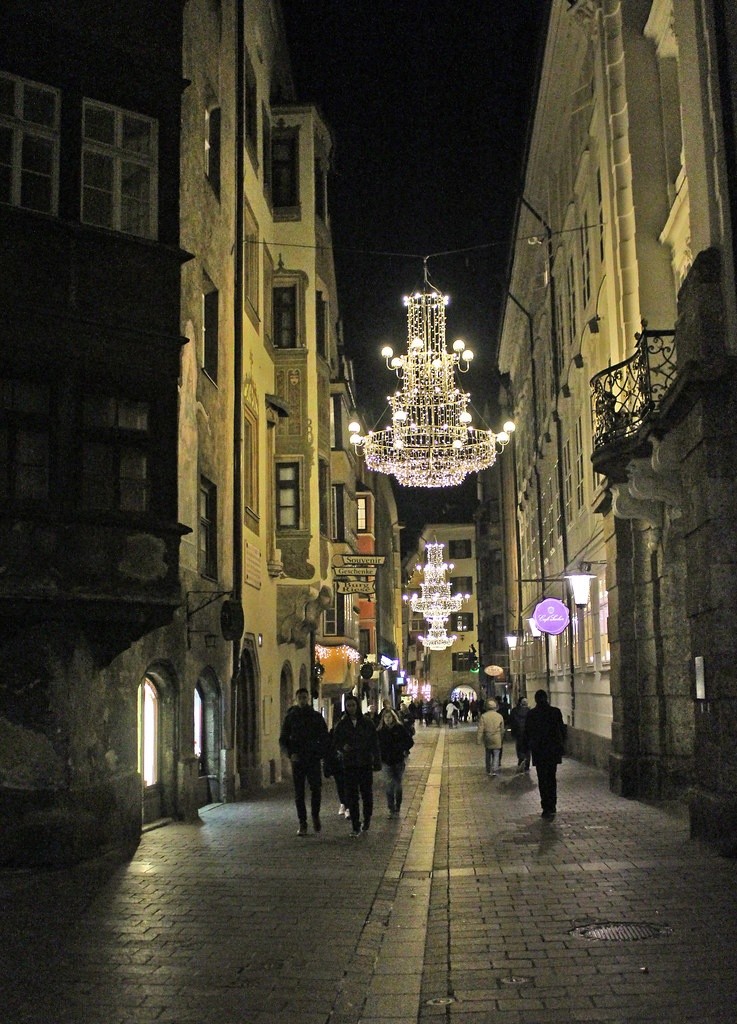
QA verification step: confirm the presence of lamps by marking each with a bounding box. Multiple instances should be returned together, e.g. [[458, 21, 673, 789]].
[[561, 358, 574, 398], [587, 274, 607, 334], [573, 319, 590, 369], [526, 617, 542, 639], [551, 391, 561, 424], [517, 491, 530, 512], [402, 536, 470, 651], [563, 559, 606, 610], [504, 630, 520, 651], [529, 436, 545, 488], [346, 257, 517, 489], [543, 424, 552, 443]]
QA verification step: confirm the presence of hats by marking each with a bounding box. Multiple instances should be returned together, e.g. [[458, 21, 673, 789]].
[[521, 698, 529, 706], [534, 689, 548, 703]]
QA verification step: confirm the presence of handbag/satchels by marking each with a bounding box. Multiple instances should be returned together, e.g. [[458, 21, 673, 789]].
[[321, 729, 334, 778], [352, 742, 374, 777], [410, 727, 416, 735]]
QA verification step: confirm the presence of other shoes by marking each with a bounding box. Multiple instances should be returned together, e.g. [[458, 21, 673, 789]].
[[311, 811, 321, 832], [296, 821, 308, 836], [543, 809, 557, 822], [349, 829, 361, 837], [393, 810, 399, 818], [386, 810, 394, 819], [362, 820, 370, 830]]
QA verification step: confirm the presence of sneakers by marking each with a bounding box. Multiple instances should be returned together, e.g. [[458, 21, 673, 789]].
[[344, 808, 351, 819], [338, 804, 345, 814]]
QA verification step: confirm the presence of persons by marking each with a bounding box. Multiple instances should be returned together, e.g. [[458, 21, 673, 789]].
[[279, 688, 329, 836], [377, 698, 415, 819], [470, 696, 511, 722], [365, 704, 378, 721], [510, 696, 531, 772], [527, 690, 565, 817], [477, 696, 508, 776], [323, 696, 382, 837], [408, 691, 469, 728]]
